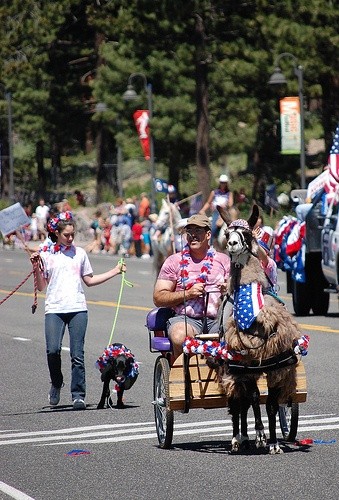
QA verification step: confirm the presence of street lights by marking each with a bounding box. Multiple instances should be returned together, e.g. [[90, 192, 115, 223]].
[[269, 52, 306, 191], [121, 72, 157, 215]]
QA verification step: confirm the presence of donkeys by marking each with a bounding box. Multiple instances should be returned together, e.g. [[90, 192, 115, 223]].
[[214, 204, 300, 456], [96, 342, 140, 409]]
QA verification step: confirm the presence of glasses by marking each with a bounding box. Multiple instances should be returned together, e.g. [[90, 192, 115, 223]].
[[185, 229, 204, 234]]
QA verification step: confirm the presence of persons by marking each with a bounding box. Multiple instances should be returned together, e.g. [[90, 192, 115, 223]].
[[89, 188, 189, 258], [198, 175, 233, 243], [153, 214, 231, 358], [30, 211, 127, 409], [35, 196, 50, 238]]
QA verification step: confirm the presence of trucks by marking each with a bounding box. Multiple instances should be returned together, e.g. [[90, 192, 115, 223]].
[[291, 168, 339, 315]]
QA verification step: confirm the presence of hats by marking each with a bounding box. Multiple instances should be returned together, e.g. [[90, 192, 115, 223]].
[[219, 175, 228, 182], [184, 215, 212, 231]]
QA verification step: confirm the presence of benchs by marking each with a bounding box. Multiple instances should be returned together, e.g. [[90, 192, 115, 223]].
[[146, 306, 172, 352]]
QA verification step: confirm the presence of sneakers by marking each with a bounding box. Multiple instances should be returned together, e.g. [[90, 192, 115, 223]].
[[48, 382, 65, 405], [73, 399, 86, 409]]
